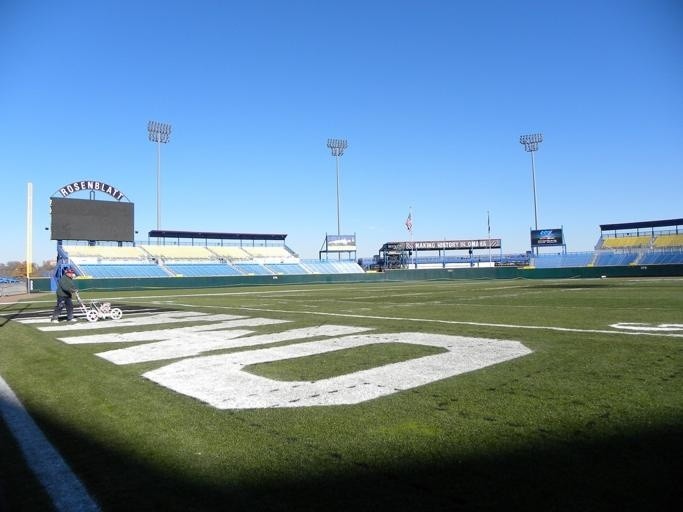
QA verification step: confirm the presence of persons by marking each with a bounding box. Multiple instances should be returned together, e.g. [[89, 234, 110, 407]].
[[51, 269, 77, 323]]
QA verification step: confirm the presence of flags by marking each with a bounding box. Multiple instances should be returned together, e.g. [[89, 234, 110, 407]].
[[405, 212, 413, 230]]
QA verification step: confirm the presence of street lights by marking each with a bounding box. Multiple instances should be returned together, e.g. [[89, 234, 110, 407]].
[[518, 133, 545, 255], [325, 138, 350, 236], [146, 121, 172, 244]]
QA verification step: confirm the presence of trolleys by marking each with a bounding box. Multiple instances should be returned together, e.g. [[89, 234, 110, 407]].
[[72, 289, 122, 321]]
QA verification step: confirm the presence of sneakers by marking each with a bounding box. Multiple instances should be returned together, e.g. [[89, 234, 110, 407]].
[[51, 320, 60, 323], [71, 319, 78, 322]]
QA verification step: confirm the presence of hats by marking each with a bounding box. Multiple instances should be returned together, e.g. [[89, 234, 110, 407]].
[[65, 268, 75, 273]]
